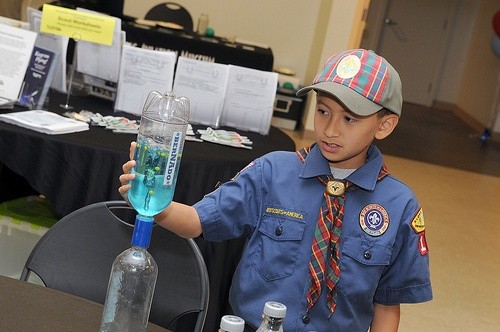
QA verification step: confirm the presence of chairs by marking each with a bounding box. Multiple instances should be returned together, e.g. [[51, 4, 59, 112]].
[[17, 199, 211, 329], [141, 2, 195, 32]]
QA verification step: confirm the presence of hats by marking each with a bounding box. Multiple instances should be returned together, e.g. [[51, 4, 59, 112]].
[[295, 47, 404, 117]]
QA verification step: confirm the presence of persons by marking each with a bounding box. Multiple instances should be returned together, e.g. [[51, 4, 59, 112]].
[[117, 47, 434, 332]]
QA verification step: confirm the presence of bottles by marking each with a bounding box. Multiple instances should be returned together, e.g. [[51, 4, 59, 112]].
[[128, 89, 190, 235], [99, 225, 159, 332], [256, 300, 286, 332], [218, 315, 245, 332]]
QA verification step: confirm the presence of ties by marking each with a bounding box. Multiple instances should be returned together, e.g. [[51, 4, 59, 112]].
[[295, 146, 392, 321]]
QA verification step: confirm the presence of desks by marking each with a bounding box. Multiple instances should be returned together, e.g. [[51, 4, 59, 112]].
[[105, 10, 275, 73], [1, 84, 299, 234], [0, 274, 179, 332]]
